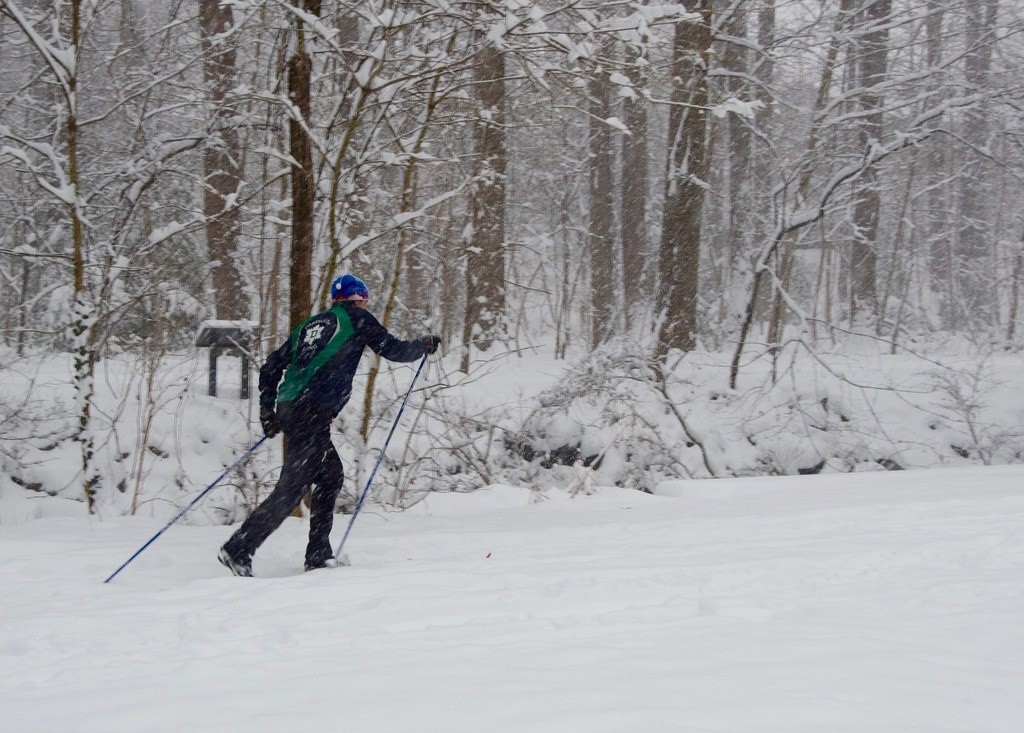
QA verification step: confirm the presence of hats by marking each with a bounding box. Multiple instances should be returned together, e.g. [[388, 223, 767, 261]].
[[330, 275, 368, 303]]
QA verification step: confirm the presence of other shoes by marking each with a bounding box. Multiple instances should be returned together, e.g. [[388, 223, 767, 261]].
[[218, 547, 254, 578], [304, 557, 351, 572]]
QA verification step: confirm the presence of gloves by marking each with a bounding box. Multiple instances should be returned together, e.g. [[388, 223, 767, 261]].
[[422, 334, 442, 356], [263, 418, 280, 439]]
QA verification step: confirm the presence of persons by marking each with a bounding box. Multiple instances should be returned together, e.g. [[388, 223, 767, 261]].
[[217, 274, 442, 577]]
